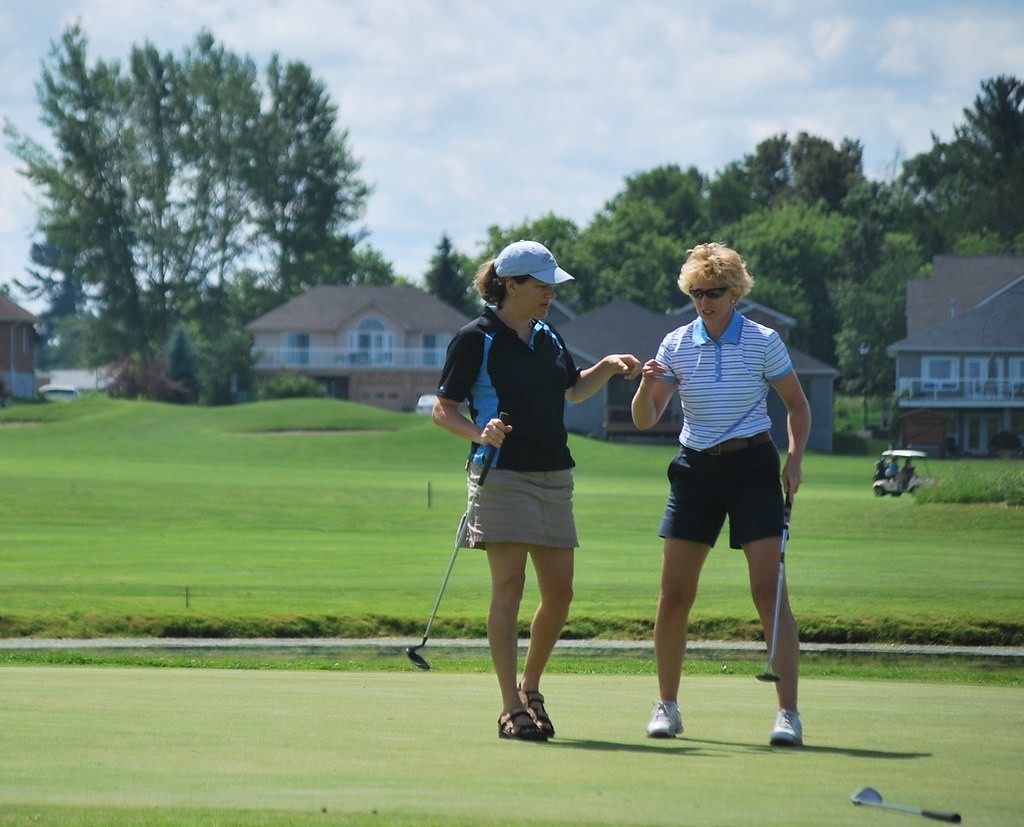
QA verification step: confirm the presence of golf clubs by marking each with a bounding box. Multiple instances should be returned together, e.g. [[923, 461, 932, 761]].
[[852, 787, 962, 824], [754, 491, 791, 683], [405, 412, 510, 670]]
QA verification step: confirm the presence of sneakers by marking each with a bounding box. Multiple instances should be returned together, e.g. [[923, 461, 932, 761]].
[[646, 699, 684, 739], [770, 710, 801, 746]]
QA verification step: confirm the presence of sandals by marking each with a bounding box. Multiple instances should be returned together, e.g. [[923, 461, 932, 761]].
[[517, 684, 555, 737], [497, 711, 545, 740]]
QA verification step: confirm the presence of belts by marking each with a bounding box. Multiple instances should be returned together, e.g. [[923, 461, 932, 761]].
[[702, 431, 772, 456]]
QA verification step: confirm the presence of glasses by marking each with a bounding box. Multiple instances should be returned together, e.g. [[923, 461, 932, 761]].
[[689, 287, 728, 300]]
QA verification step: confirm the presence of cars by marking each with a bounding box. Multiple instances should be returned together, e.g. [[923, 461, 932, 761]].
[[37, 383, 81, 402], [415, 393, 435, 414]]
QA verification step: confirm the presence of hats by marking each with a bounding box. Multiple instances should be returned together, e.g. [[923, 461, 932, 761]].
[[493, 240, 575, 284]]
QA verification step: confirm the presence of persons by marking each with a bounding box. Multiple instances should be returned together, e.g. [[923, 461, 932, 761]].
[[902, 458, 912, 473], [875, 457, 899, 481], [631, 242, 811, 747], [432, 240, 642, 742]]
